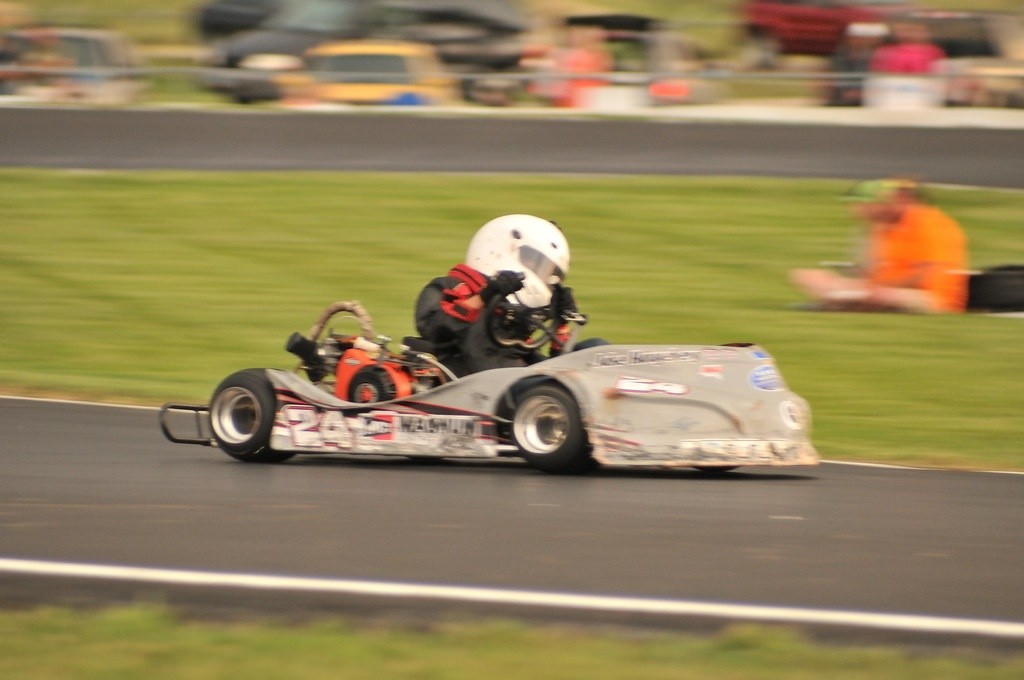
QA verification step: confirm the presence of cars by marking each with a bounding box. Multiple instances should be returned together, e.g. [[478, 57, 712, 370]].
[[1, 2, 1023, 114]]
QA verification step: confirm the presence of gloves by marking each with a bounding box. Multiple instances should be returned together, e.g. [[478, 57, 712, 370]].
[[550, 285, 577, 325], [479, 270, 523, 306]]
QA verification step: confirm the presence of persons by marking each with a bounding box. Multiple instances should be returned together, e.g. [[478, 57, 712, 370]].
[[826, 19, 947, 108], [789, 178, 1024, 314], [519, 25, 615, 107], [414, 213, 611, 387]]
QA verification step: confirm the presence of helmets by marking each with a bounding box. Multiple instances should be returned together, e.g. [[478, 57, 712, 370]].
[[465, 214, 569, 308]]
